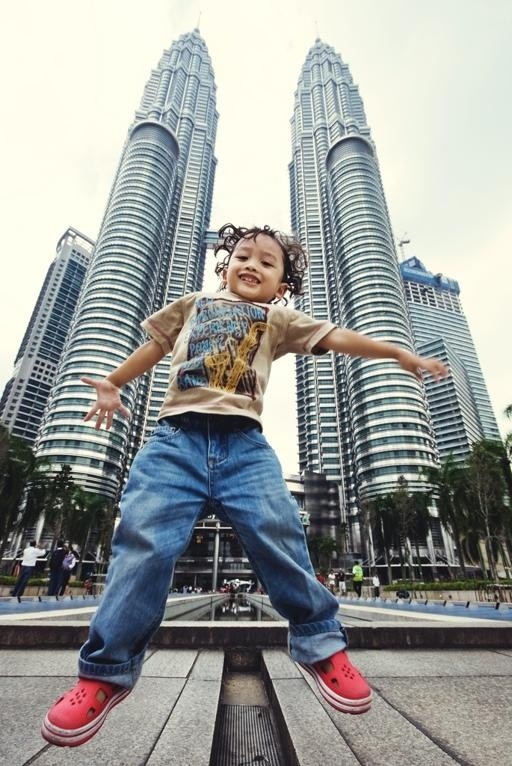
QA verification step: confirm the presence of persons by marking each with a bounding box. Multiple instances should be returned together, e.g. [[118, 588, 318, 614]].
[[317, 560, 346, 595], [10, 539, 93, 597], [352, 561, 364, 597], [172, 580, 241, 595], [43, 221, 446, 748], [372, 573, 380, 598]]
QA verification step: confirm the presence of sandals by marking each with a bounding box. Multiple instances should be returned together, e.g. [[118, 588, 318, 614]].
[[41, 679, 130, 747], [296, 654, 373, 714]]
